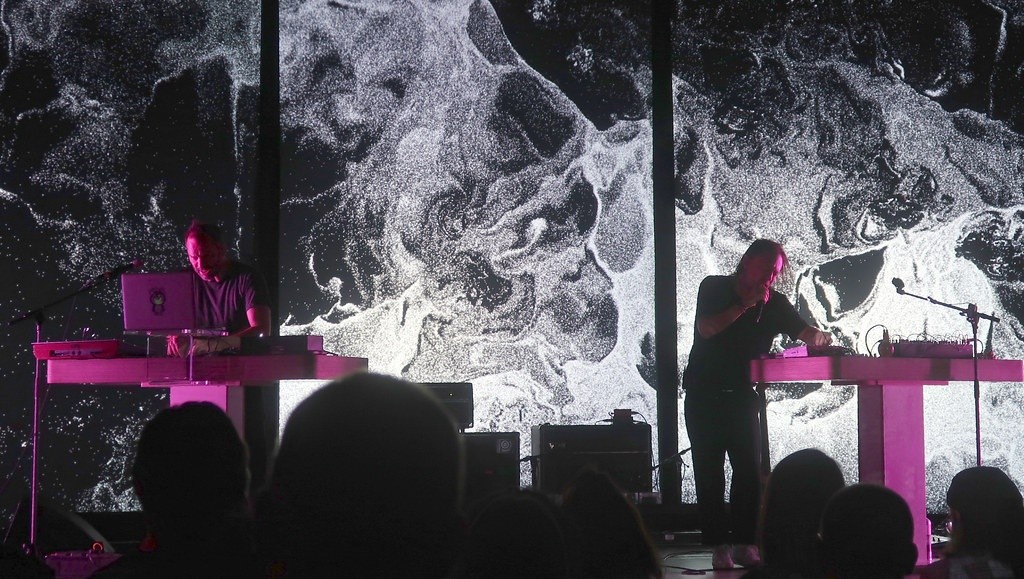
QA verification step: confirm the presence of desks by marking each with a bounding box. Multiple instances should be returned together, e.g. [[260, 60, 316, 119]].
[[49, 353, 369, 432], [751, 356, 1024, 567]]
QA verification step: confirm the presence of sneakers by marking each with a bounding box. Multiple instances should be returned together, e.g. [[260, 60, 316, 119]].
[[712, 544, 760, 569]]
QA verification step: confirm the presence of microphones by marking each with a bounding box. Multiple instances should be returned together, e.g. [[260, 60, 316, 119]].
[[753, 301, 764, 322], [97, 259, 142, 280]]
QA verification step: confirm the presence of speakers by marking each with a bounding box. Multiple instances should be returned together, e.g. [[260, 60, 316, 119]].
[[463, 431, 520, 502], [532, 425, 653, 497]]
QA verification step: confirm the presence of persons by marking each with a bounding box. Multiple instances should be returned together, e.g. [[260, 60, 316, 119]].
[[0, 372, 1024, 579], [166, 221, 272, 494], [682, 240, 833, 570]]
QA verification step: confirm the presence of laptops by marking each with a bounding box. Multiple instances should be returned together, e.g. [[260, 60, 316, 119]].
[[122, 272, 229, 338]]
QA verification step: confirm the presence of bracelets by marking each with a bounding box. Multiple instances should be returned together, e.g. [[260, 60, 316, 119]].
[[734, 299, 749, 312]]
